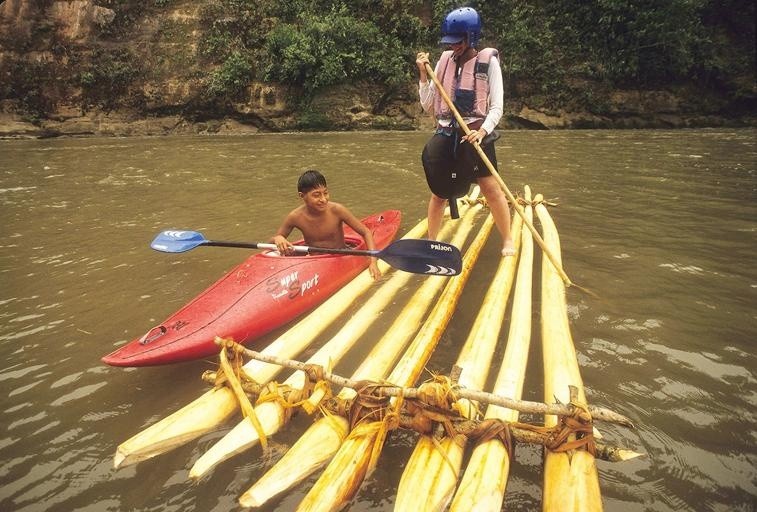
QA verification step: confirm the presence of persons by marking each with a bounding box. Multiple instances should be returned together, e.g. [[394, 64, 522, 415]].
[[415, 5, 517, 257], [266, 169, 383, 282]]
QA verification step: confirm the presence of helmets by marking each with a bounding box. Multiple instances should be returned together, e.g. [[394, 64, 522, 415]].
[[439, 7, 481, 48]]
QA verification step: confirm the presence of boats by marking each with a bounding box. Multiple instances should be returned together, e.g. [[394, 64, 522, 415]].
[[114, 180, 643, 511], [101, 211, 402, 366]]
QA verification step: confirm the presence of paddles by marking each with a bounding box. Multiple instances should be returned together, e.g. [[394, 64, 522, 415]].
[[151, 229, 462, 275]]
